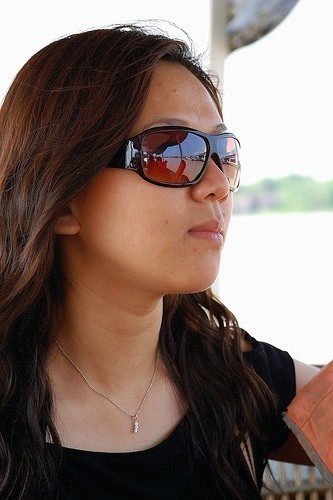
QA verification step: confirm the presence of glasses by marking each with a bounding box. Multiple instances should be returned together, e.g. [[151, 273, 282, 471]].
[[104, 125, 242, 192]]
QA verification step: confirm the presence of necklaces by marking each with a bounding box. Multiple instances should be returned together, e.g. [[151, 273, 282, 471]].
[[52, 334, 160, 434]]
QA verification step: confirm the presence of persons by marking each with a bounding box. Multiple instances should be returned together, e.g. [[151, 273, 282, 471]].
[[1, 19, 333, 500]]
